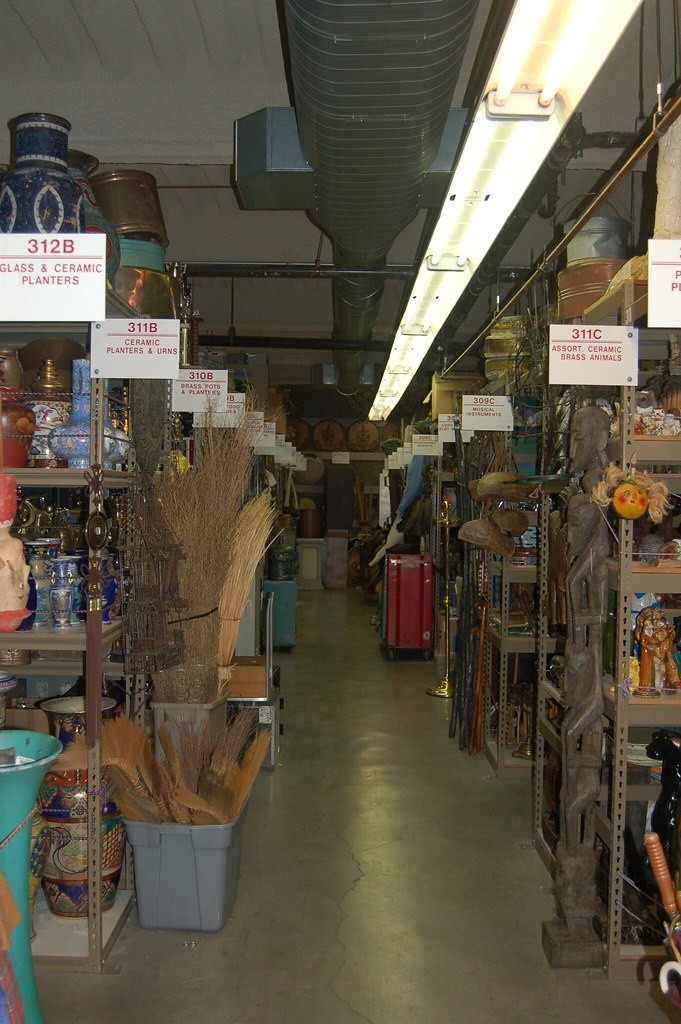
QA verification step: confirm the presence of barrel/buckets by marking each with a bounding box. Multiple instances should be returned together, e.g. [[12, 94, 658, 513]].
[[563, 192, 633, 267], [89, 169, 169, 249]]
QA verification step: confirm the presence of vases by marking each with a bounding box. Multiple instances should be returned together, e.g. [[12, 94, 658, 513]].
[[0, 113, 84, 235], [0, 347, 128, 1024], [65, 150, 119, 283]]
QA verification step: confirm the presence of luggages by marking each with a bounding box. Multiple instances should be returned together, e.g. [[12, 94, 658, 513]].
[[380, 546, 433, 661]]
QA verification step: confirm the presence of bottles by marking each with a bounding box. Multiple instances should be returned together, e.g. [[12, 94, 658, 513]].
[[25, 538, 117, 629], [0, 112, 120, 280]]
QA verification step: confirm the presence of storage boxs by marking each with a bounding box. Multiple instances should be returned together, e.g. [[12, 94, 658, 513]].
[[225, 656, 266, 698], [122, 789, 247, 932], [263, 581, 295, 647], [430, 369, 481, 422], [150, 691, 227, 763], [4, 696, 53, 735]]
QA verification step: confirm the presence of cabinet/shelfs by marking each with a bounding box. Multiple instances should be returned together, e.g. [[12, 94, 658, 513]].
[[0, 324, 172, 972], [427, 277, 681, 982]]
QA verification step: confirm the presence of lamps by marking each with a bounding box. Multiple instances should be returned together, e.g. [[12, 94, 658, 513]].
[[368, 0, 646, 421]]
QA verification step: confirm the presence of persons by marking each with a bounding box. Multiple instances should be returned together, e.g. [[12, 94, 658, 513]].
[[543, 406, 610, 968], [634, 406, 681, 435], [638, 616, 681, 694]]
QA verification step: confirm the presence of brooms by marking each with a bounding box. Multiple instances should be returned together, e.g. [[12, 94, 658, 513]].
[[46, 705, 275, 827], [136, 385, 285, 702]]
